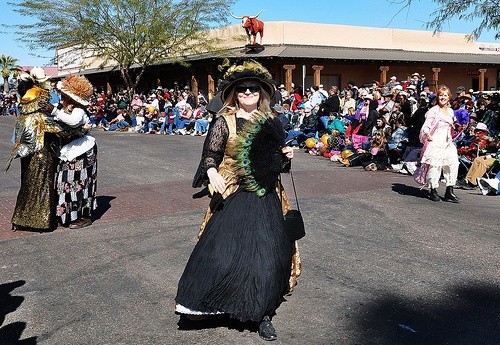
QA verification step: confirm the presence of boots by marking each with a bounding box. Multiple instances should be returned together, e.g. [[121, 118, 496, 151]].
[[430, 188, 441, 201], [444, 186, 459, 202]]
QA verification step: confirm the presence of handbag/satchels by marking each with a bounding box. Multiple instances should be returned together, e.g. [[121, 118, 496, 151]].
[[285, 209, 306, 241]]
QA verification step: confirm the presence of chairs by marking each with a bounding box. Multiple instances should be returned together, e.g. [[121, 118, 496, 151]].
[[459, 144, 497, 179]]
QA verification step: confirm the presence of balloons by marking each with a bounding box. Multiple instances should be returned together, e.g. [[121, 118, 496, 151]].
[[342, 149, 351, 158], [321, 133, 330, 146], [306, 138, 316, 149], [148, 106, 155, 113]]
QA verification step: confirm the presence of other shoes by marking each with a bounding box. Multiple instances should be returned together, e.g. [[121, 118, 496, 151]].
[[476, 177, 499, 196], [258, 315, 277, 341], [453, 178, 476, 190], [391, 164, 409, 175], [69, 217, 93, 229], [134, 128, 208, 137], [337, 157, 350, 167]]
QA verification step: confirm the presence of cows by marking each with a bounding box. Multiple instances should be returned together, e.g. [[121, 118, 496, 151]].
[[229, 10, 264, 47]]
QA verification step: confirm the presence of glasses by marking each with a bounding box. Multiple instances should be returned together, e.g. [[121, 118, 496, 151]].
[[421, 76, 425, 78], [236, 85, 260, 93]]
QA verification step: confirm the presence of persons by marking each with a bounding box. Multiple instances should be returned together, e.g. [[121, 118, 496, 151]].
[[174, 60, 294, 341], [0, 74, 500, 229]]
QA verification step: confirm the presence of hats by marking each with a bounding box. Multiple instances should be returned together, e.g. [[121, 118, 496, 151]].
[[456, 85, 471, 99], [474, 122, 491, 135], [216, 57, 275, 106], [470, 112, 477, 119], [277, 72, 427, 129], [56, 74, 93, 106], [19, 86, 50, 114], [150, 86, 191, 100]]
